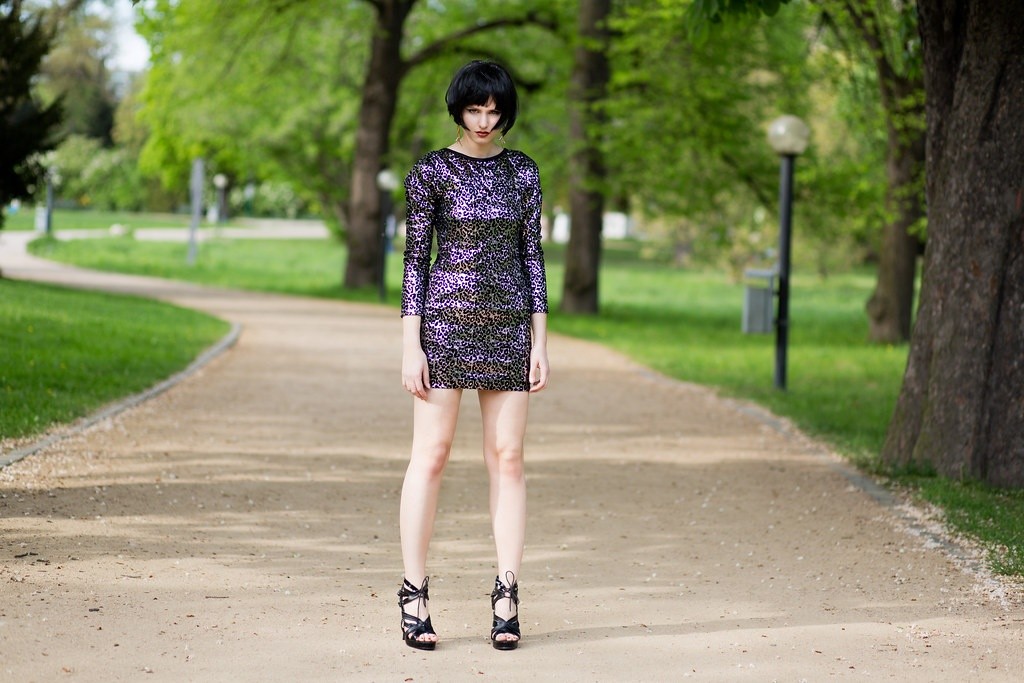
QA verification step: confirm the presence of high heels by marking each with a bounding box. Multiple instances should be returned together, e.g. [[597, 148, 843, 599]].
[[490, 570, 521, 649], [397, 576, 438, 650]]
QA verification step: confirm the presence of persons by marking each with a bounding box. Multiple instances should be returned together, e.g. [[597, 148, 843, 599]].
[[396, 61, 550, 651]]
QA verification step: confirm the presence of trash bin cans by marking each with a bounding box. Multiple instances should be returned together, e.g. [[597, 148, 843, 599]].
[[743, 270, 777, 335]]
